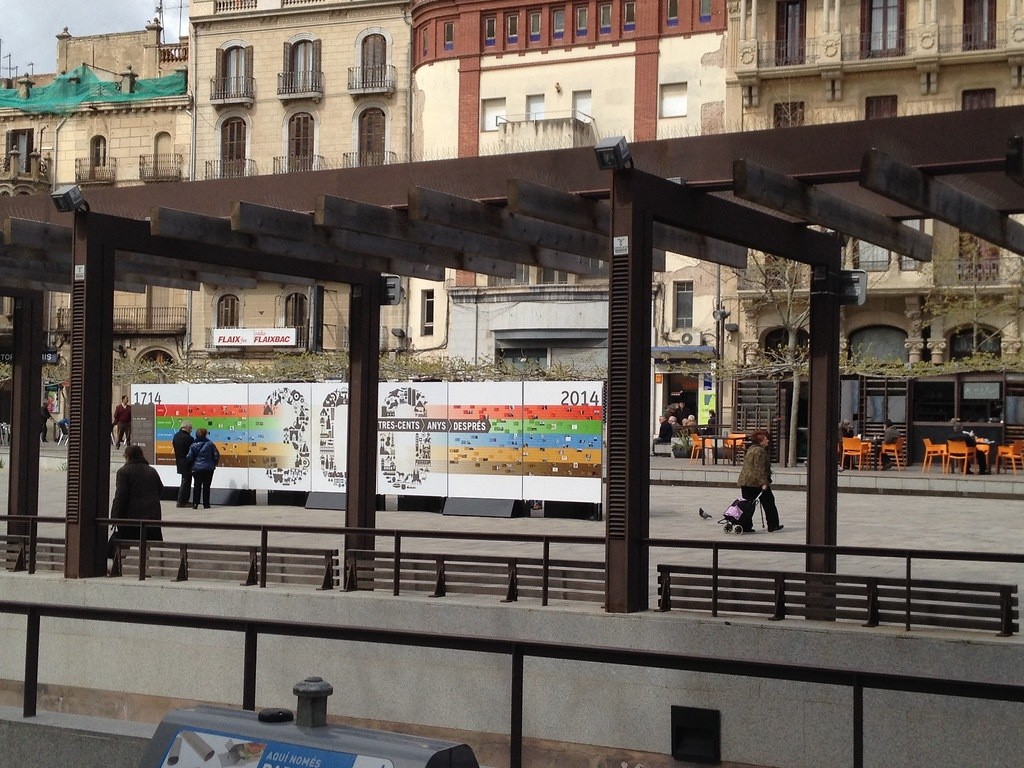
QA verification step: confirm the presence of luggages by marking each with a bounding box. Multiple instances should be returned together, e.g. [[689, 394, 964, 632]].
[[718, 489, 768, 535]]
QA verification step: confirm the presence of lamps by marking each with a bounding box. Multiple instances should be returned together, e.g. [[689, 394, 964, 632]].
[[50, 185, 90, 213], [725, 323, 739, 332], [594, 135, 634, 171], [391, 328, 405, 339]]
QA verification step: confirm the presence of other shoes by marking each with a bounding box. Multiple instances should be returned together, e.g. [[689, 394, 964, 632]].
[[178, 502, 193, 507], [966, 470, 974, 475], [844, 464, 858, 469], [193, 503, 198, 509], [116, 443, 120, 449], [881, 462, 894, 471], [203, 504, 210, 508], [978, 471, 989, 475]]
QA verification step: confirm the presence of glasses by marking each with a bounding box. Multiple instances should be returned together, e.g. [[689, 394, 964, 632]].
[[123, 454, 128, 458]]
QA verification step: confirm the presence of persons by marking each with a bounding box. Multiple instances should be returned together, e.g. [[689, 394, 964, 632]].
[[111, 445, 166, 578], [738, 430, 784, 532], [873, 420, 900, 470], [113, 395, 131, 450], [949, 421, 989, 476], [172, 422, 198, 508], [40, 402, 57, 442], [186, 428, 220, 508], [837, 419, 858, 470], [650, 402, 715, 455]]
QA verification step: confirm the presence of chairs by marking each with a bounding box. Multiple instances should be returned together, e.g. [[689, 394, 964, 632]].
[[690, 433, 713, 465], [995, 440, 1024, 476], [946, 440, 977, 475], [878, 438, 906, 471], [921, 438, 948, 474], [841, 437, 869, 471], [722, 434, 747, 465], [57, 430, 69, 446]]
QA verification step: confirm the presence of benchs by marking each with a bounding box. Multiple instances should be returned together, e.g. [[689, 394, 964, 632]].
[[340, 548, 606, 610], [107, 539, 339, 591], [653, 563, 1019, 638], [0, 535, 65, 573]]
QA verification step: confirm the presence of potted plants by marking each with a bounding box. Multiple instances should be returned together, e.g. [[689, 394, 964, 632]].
[[671, 428, 693, 459]]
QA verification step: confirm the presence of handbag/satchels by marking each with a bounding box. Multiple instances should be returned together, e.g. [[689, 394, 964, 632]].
[[182, 459, 195, 477], [106, 526, 121, 559]]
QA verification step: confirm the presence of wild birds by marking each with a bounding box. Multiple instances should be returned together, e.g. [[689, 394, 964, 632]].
[[699, 508, 712, 520]]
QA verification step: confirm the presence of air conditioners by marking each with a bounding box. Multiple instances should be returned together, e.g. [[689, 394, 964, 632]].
[[680, 330, 703, 346]]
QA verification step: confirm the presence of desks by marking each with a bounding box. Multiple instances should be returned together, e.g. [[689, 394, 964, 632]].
[[698, 436, 749, 466], [846, 439, 885, 471], [958, 444, 991, 474]]
